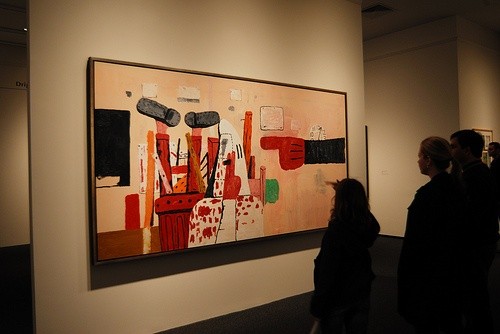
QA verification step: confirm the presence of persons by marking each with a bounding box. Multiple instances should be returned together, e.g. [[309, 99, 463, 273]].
[[309, 177, 380, 334], [447, 129, 494, 333], [395, 135, 468, 334], [487, 142, 500, 180]]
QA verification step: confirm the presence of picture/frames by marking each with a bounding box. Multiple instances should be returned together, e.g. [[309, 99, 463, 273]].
[[472, 128, 494, 170], [86, 56, 349, 267]]
